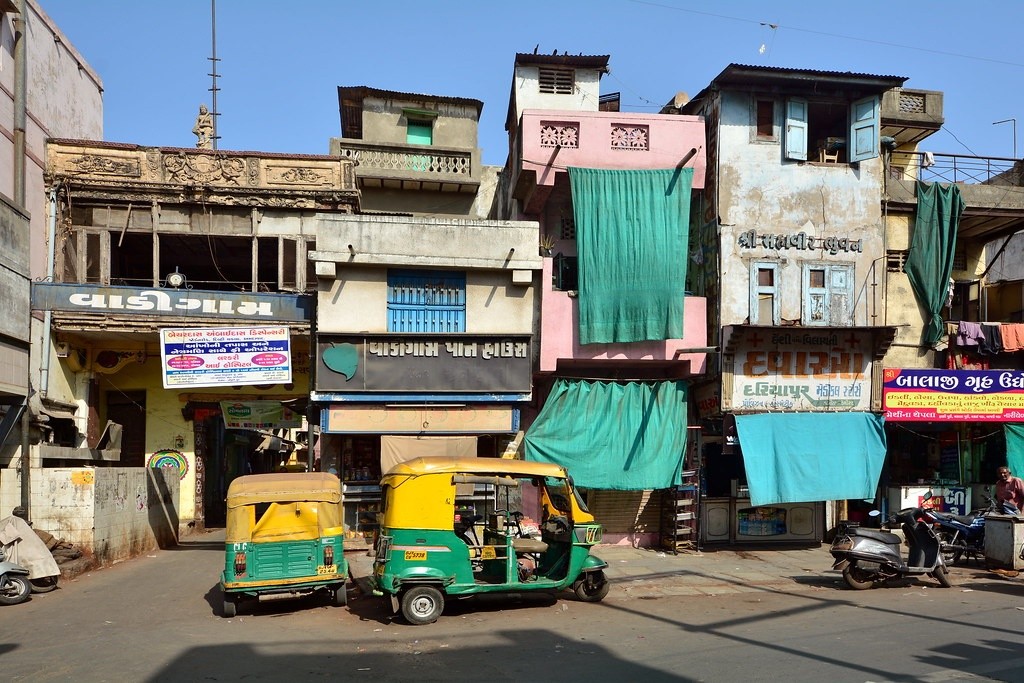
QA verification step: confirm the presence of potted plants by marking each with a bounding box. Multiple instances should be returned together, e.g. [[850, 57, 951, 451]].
[[539, 233, 558, 258]]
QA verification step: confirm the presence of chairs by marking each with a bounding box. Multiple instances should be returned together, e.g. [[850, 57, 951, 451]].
[[490, 513, 548, 580]]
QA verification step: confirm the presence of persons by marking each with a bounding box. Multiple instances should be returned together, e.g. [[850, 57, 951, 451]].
[[192, 105, 214, 145], [996, 466, 1024, 515]]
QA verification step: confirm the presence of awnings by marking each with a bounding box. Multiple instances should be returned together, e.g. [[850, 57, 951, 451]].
[[721, 324, 911, 359], [714, 63, 909, 95]]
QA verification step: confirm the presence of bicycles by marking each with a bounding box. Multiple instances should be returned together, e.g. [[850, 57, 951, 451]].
[[454, 509, 541, 570]]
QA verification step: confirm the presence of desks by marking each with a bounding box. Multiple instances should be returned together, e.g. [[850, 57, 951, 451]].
[[983, 516, 1024, 571], [888, 485, 971, 517]]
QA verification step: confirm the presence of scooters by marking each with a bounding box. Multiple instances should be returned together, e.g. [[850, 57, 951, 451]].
[[829, 491, 951, 590]]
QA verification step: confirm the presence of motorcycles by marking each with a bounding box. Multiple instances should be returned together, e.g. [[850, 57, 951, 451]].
[[924, 486, 1019, 566], [0, 516, 58, 605], [373, 457, 610, 625], [220, 472, 350, 617]]
